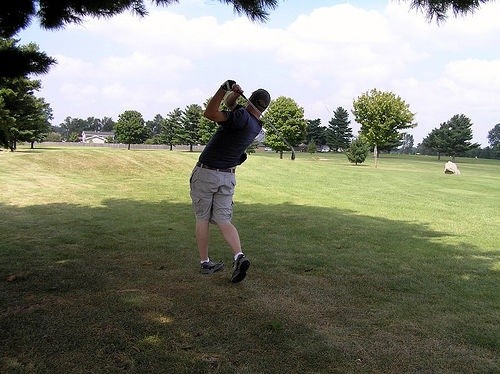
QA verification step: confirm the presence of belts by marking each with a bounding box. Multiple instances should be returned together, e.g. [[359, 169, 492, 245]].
[[196, 162, 236, 173]]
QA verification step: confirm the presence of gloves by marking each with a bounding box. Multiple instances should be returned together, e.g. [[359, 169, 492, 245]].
[[221, 79, 236, 91]]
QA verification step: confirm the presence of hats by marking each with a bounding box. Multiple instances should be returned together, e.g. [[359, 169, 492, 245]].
[[252, 89, 271, 109]]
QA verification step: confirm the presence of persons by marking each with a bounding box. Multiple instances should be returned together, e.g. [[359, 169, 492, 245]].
[[189, 80, 271, 282]]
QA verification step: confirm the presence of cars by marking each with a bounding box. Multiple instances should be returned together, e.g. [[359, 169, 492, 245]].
[[317, 146, 344, 153]]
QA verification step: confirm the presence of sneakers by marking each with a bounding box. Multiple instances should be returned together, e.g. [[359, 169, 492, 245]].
[[201, 260, 224, 274], [231, 255, 251, 283]]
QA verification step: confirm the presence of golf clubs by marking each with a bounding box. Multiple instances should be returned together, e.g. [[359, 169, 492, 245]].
[[239, 90, 295, 161]]
